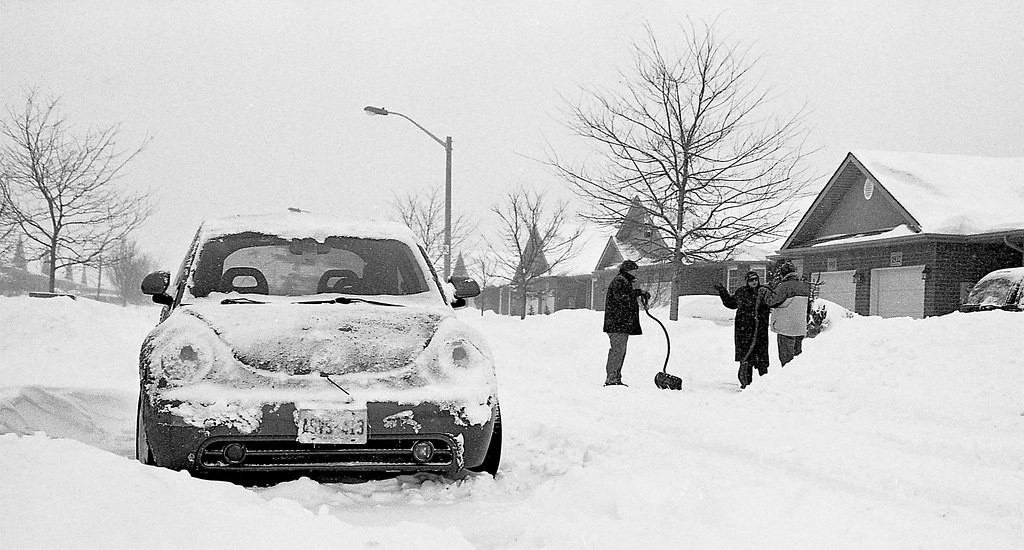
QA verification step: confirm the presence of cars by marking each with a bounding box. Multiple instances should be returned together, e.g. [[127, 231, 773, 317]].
[[135, 210, 501, 480]]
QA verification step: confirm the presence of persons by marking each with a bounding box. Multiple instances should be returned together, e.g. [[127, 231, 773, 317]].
[[603, 260, 651, 387], [757, 259, 810, 367], [712, 271, 778, 388]]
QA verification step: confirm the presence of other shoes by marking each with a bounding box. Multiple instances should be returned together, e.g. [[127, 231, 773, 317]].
[[605, 381, 628, 387]]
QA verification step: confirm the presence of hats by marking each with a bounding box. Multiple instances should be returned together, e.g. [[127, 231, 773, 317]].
[[780, 262, 796, 276], [620, 260, 638, 271], [746, 271, 758, 283]]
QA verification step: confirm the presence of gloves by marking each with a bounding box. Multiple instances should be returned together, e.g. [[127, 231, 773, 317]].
[[714, 283, 728, 294], [755, 285, 763, 291], [638, 287, 651, 299]]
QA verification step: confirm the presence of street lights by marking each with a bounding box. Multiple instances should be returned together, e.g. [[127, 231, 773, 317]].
[[363, 106, 455, 284]]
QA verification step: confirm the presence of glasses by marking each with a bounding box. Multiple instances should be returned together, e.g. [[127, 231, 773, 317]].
[[747, 276, 759, 282]]
